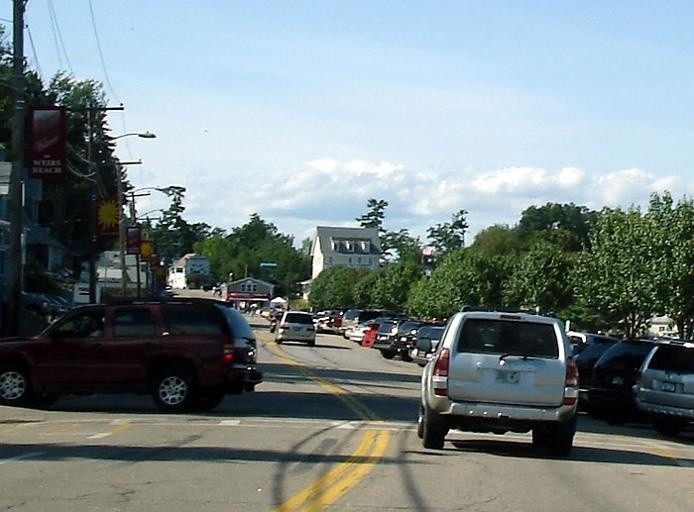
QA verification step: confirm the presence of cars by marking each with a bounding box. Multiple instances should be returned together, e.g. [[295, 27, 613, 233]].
[[1, 297, 263, 413]]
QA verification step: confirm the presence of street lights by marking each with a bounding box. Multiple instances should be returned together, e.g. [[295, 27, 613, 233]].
[[112, 132, 158, 140]]
